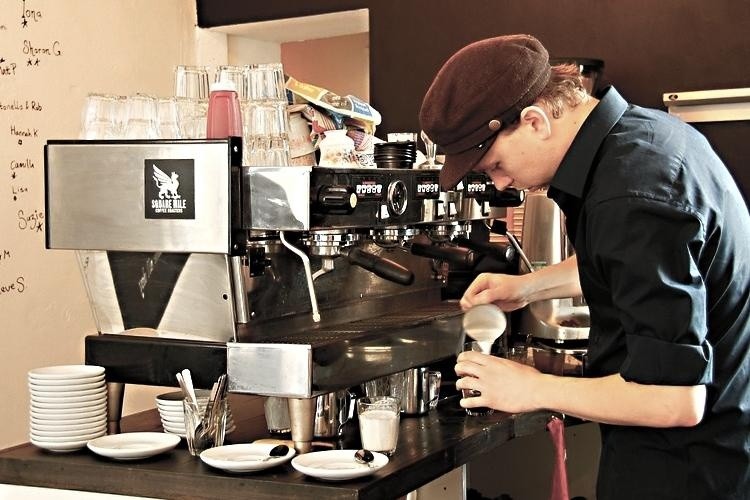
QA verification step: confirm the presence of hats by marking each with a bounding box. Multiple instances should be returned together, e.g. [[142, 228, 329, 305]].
[[418, 32, 553, 192]]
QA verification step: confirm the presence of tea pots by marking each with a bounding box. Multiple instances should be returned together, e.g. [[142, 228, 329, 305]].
[[455, 300, 511, 363]]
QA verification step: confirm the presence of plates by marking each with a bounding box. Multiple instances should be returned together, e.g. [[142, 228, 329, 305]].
[[26, 364, 109, 454], [199, 443, 296, 473], [290, 448, 388, 485], [82, 430, 181, 463], [155, 389, 237, 441]]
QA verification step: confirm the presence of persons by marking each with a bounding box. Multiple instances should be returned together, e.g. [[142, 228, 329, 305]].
[[418, 34, 749, 500]]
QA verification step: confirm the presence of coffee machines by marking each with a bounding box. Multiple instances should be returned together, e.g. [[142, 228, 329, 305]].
[[43, 139, 528, 427], [518, 190, 592, 345]]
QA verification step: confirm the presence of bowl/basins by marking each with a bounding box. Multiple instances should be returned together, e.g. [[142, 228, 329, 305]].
[[373, 141, 418, 170]]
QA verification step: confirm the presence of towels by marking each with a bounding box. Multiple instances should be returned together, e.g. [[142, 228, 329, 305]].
[[547, 417, 570, 500]]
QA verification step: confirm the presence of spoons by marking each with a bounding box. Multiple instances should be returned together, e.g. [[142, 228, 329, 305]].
[[354, 447, 378, 471], [175, 366, 229, 457], [262, 443, 288, 462]]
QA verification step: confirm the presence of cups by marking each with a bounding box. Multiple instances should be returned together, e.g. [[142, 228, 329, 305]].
[[314, 388, 356, 439], [458, 341, 508, 417], [358, 367, 443, 416], [182, 398, 227, 458], [355, 394, 401, 459], [81, 61, 363, 170], [260, 395, 292, 435]]
